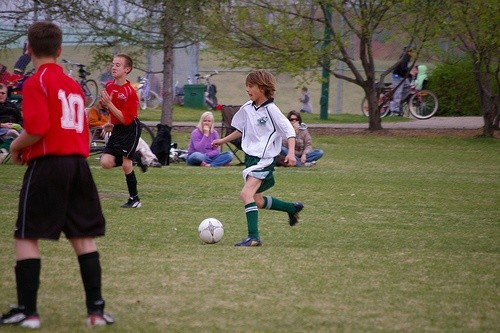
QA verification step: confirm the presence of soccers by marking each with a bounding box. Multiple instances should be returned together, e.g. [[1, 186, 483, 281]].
[[197, 217, 224, 244]]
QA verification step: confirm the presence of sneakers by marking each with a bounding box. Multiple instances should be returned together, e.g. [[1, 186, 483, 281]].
[[135, 151, 147, 171], [121, 196, 140, 209], [288, 201, 303, 225], [235, 236, 261, 247]]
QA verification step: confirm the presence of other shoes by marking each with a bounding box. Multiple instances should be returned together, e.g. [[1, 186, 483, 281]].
[[85, 313, 114, 328], [0, 308, 40, 329], [149, 160, 162, 167], [200, 161, 206, 166]]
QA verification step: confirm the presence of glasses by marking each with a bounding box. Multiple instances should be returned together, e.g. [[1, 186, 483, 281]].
[[290, 118, 299, 121]]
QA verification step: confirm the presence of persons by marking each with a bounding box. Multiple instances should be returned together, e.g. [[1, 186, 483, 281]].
[[186, 111, 235, 166], [87, 96, 163, 168], [0, 22, 116, 329], [210, 70, 304, 247], [0, 83, 24, 128], [276, 110, 323, 168], [390, 49, 414, 116], [412, 64, 429, 93], [100, 54, 142, 208]]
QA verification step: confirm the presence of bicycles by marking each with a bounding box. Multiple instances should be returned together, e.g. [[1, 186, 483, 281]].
[[62, 58, 99, 108], [360, 71, 440, 121], [176, 70, 218, 111], [132, 66, 160, 112]]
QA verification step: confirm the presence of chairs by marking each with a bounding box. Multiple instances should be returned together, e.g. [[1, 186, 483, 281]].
[[85, 108, 111, 146], [221, 105, 246, 164]]
